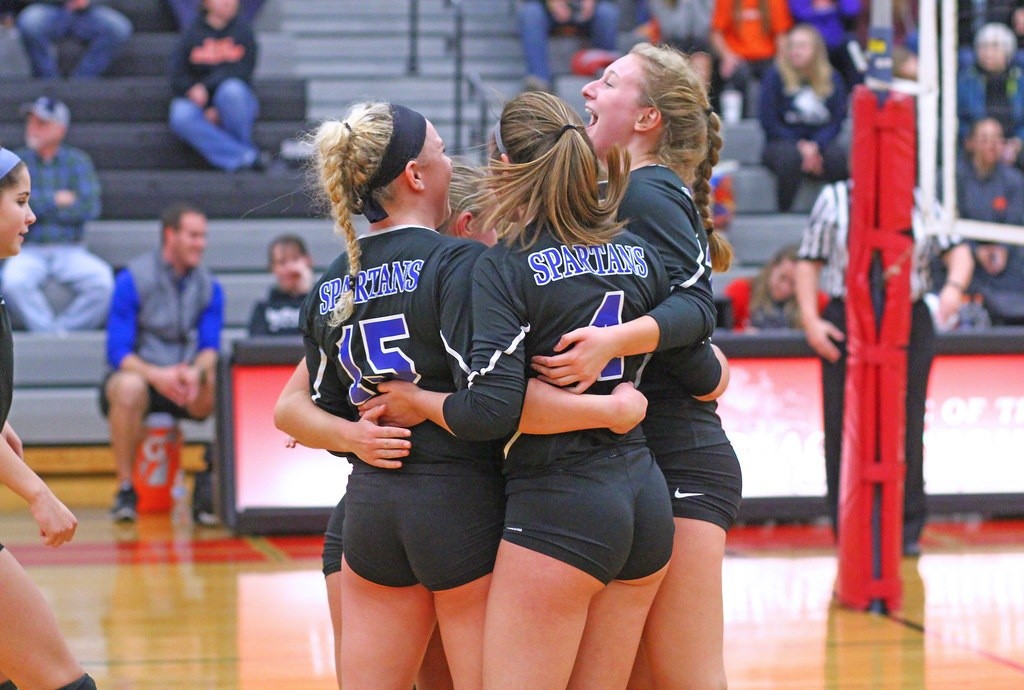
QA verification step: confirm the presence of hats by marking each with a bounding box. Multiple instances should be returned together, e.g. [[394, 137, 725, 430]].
[[22, 96, 70, 127]]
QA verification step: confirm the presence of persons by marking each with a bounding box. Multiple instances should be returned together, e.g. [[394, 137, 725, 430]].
[[0, 0, 1023, 690], [98, 204, 229, 525]]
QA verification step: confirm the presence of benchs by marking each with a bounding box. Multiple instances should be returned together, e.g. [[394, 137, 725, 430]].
[[0, 0, 824, 445]]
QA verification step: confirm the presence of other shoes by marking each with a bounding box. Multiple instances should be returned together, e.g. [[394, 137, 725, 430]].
[[904, 541, 921, 558], [524, 75, 548, 94], [109, 486, 138, 523], [251, 150, 272, 173]]
